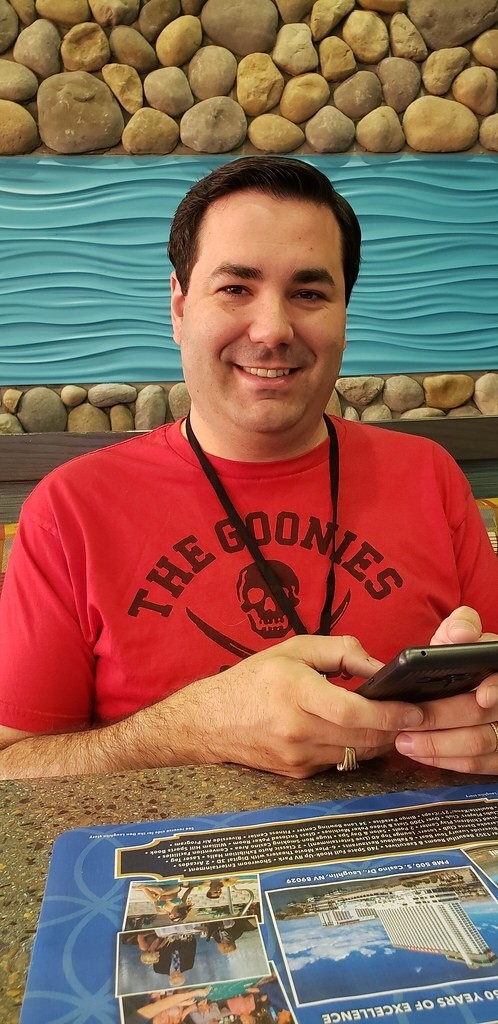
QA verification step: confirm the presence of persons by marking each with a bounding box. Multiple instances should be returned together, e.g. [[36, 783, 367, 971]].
[[206, 877, 239, 899], [124, 972, 294, 1024], [139, 882, 195, 923], [137, 922, 244, 986], [0, 158, 498, 779]]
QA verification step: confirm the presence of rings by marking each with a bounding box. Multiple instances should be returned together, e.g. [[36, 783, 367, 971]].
[[336, 748, 359, 770]]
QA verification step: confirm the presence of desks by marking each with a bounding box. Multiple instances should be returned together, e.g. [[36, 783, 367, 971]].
[[1, 758, 497, 1024]]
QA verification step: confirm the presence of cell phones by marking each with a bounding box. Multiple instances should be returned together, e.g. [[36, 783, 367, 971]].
[[353, 641, 498, 702]]
